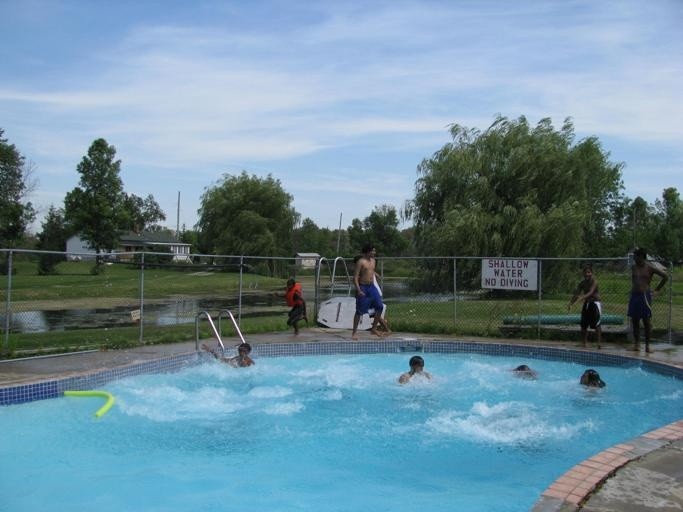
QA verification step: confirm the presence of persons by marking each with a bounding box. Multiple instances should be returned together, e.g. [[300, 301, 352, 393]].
[[201, 343, 256, 370], [398, 354, 432, 386], [354, 253, 392, 335], [626, 248, 669, 352], [512, 364, 537, 381], [579, 368, 605, 390], [566, 263, 603, 351], [275, 279, 309, 336], [351, 245, 383, 342]]
